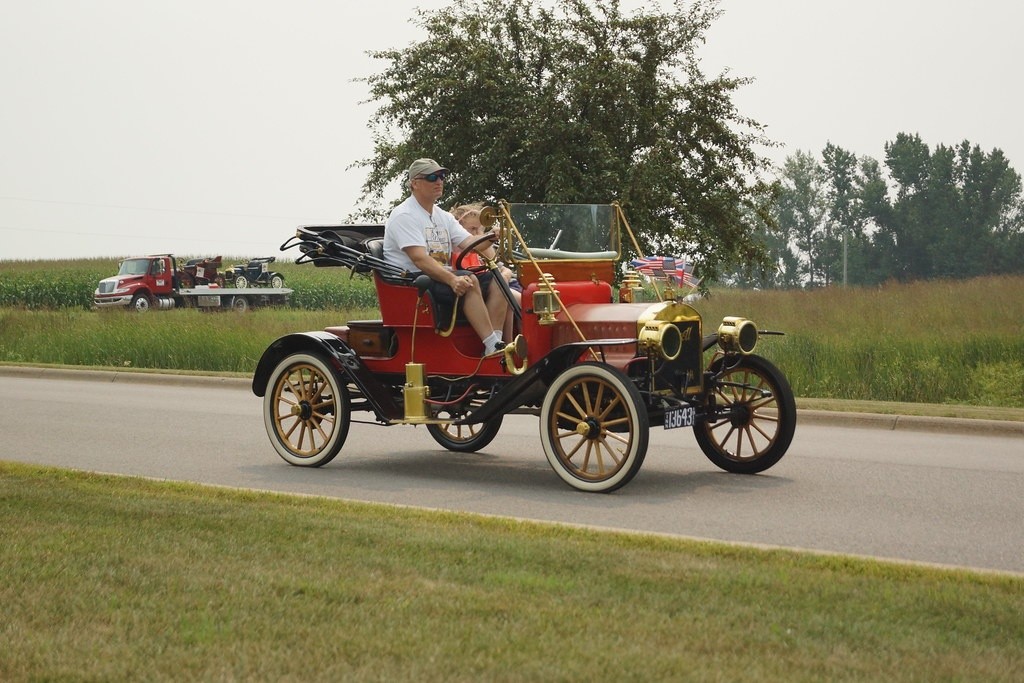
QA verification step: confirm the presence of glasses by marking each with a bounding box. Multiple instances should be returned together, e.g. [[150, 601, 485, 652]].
[[415, 172, 445, 182]]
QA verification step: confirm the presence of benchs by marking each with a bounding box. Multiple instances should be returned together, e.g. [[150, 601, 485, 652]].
[[360, 236, 475, 337], [183, 256, 222, 269]]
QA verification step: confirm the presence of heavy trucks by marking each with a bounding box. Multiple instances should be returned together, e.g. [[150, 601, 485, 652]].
[[93, 253, 295, 314]]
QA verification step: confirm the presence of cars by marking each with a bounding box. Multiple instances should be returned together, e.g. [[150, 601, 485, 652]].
[[224, 256, 286, 290], [170, 254, 227, 290], [252, 198, 797, 495]]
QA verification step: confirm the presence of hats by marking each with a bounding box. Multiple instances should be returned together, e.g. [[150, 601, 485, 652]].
[[409, 158, 451, 180]]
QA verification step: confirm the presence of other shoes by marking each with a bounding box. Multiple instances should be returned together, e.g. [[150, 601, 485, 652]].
[[482, 341, 507, 357]]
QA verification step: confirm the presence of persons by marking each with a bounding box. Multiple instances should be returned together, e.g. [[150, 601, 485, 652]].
[[383, 159, 511, 355], [449, 201, 524, 344]]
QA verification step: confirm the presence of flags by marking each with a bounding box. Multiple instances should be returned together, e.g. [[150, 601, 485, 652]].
[[632, 256, 699, 289]]
